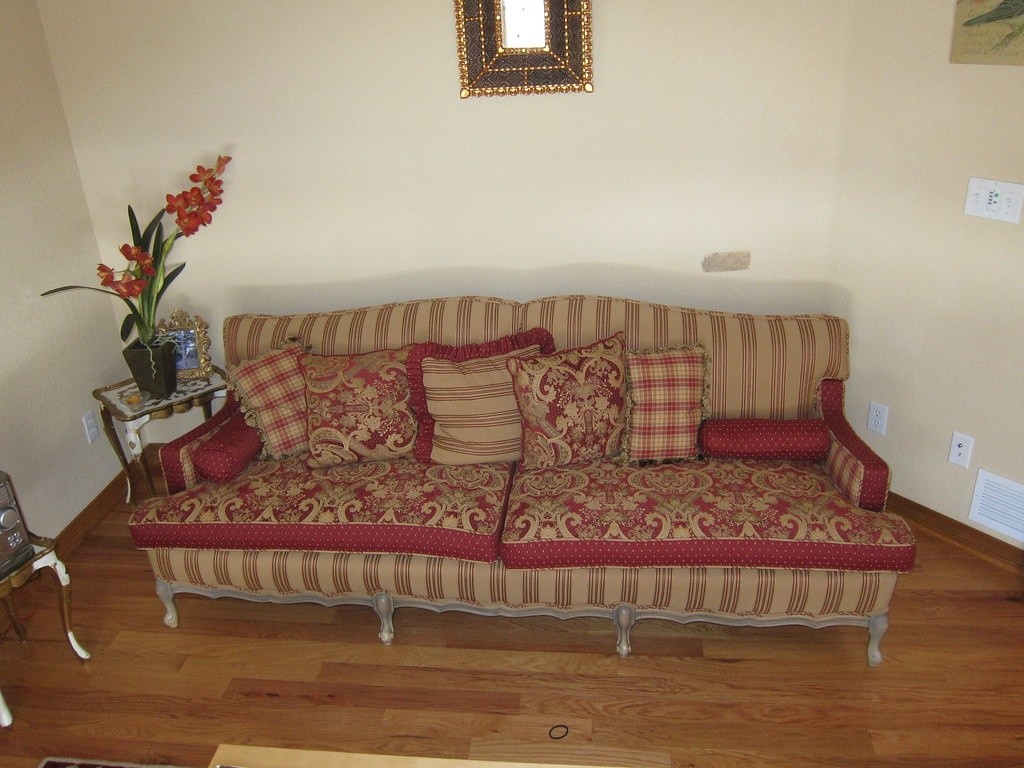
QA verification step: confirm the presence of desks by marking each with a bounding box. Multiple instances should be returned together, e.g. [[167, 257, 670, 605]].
[[92, 361, 228, 505]]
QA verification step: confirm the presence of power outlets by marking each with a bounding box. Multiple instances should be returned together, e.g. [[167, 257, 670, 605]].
[[82, 410, 100, 445], [866, 403, 889, 435]]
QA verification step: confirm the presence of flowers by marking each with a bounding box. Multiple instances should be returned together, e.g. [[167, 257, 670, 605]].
[[40, 156, 230, 379]]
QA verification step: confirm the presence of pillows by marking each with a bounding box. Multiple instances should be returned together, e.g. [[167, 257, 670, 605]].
[[617, 341, 712, 467], [508, 330, 634, 471], [228, 335, 313, 463], [194, 402, 270, 480], [409, 325, 558, 466], [298, 342, 422, 471], [694, 418, 830, 461]]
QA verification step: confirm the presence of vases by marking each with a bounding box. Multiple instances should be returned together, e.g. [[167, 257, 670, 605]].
[[122, 338, 176, 400]]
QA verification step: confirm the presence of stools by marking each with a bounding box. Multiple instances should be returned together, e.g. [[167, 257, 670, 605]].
[[0, 531, 91, 730]]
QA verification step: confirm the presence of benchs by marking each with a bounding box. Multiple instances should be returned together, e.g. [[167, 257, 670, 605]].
[[129, 293, 915, 667]]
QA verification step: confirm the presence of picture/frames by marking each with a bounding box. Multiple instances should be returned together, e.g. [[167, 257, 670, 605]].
[[153, 310, 213, 381], [948, 0, 1024, 66], [455, 1, 595, 97]]
